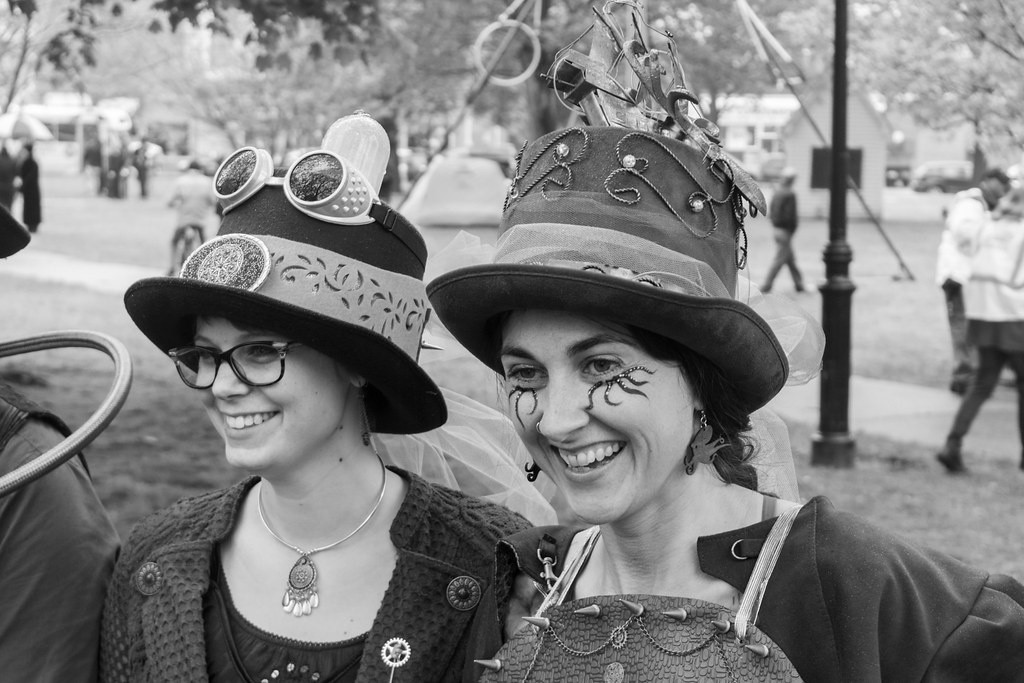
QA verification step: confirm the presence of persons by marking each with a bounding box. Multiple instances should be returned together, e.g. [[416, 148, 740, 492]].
[[934, 169, 1024, 471], [98, 147, 536, 682], [423, 120, 1024, 683], [81, 140, 152, 199], [759, 170, 803, 294], [0, 146, 123, 683], [0, 141, 42, 233]]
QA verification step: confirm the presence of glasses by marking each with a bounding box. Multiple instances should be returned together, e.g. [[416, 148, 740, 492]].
[[169, 340, 302, 388]]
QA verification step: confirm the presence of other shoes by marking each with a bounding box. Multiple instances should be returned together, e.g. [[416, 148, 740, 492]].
[[939, 450, 965, 472]]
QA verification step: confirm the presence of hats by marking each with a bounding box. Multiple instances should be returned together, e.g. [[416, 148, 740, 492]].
[[424, 2, 823, 408], [121, 144, 447, 436]]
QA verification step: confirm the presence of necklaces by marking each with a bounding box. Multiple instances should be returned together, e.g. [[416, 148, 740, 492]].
[[258, 453, 386, 617]]
